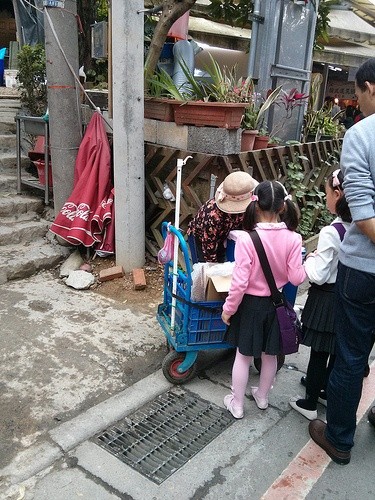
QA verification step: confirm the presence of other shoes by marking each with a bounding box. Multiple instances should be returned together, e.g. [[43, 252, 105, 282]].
[[289, 395, 318, 421], [301, 376, 307, 386], [223, 394, 244, 419], [250, 386, 268, 410], [318, 389, 327, 408]]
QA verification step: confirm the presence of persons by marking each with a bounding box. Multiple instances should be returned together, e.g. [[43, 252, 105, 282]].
[[179, 59, 375, 465]]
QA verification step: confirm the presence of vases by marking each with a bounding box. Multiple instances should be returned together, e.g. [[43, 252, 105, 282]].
[[29, 136, 54, 187], [241, 131, 258, 152]]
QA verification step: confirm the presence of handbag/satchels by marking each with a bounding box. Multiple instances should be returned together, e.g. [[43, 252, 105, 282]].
[[276, 300, 301, 355]]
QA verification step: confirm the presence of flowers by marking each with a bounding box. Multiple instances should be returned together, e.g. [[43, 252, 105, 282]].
[[234, 76, 283, 129]]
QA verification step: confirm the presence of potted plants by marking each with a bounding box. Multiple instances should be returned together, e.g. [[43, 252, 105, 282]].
[[16, 43, 50, 135], [303, 73, 346, 143], [254, 86, 310, 150], [84, 68, 108, 111], [147, 52, 250, 129], [286, 152, 336, 254], [144, 67, 175, 122]]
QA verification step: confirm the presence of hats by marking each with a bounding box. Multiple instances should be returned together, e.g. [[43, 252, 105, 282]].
[[215, 171, 260, 214]]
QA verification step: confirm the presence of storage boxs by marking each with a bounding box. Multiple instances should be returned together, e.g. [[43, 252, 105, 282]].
[[167, 273, 227, 345], [4, 69, 23, 88]]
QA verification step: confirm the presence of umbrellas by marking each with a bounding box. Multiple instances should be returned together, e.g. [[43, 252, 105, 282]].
[[49, 107, 116, 258]]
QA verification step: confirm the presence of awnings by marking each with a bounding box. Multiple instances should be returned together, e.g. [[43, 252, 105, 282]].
[[187, 16, 252, 39], [312, 7, 375, 68]]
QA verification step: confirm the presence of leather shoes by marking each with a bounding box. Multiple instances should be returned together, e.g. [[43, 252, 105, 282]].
[[309, 419, 351, 465]]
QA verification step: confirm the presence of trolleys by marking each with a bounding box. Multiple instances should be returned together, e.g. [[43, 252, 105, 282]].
[[156, 221, 298, 385]]
